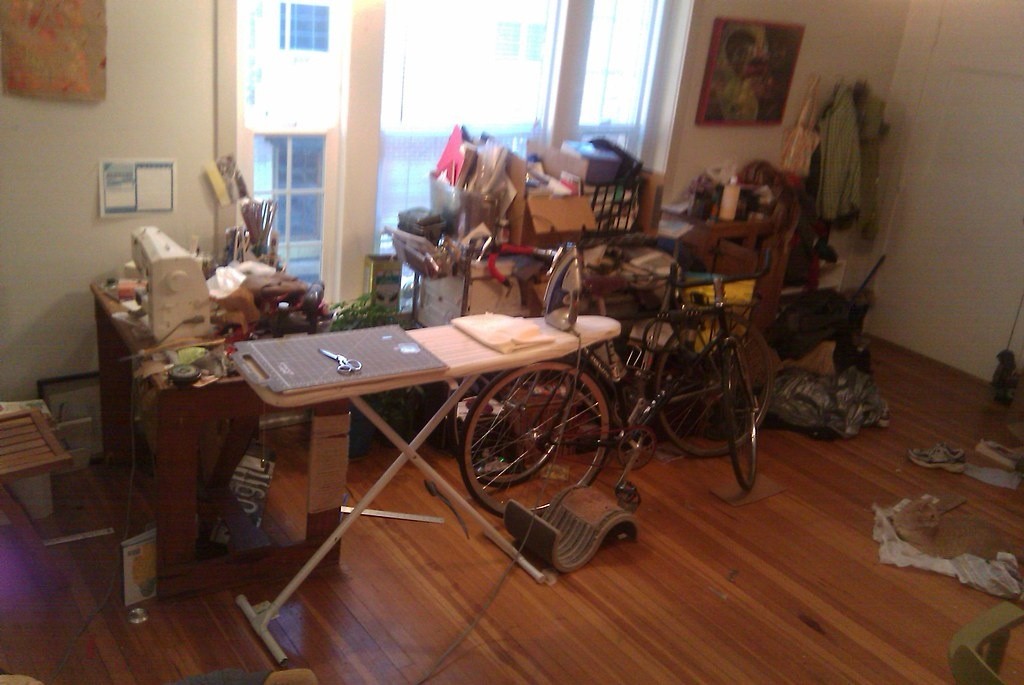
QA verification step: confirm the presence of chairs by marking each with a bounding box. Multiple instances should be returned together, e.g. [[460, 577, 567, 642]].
[[0, 410, 73, 597]]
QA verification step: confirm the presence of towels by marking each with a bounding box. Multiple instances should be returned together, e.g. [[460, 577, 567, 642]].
[[452, 312, 555, 354]]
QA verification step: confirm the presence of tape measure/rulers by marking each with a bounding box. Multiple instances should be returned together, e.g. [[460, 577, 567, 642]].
[[340, 505, 445, 525]]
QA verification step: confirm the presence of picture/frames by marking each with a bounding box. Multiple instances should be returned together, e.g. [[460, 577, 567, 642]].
[[695, 16, 805, 129]]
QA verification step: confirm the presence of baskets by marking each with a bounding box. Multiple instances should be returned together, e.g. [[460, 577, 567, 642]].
[[577, 177, 645, 238]]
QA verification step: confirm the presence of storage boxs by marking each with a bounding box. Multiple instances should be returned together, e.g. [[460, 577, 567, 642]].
[[482, 136, 597, 247]]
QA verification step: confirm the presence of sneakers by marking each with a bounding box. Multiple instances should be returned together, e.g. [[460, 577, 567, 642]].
[[877, 409, 890, 427], [907, 441, 966, 473]]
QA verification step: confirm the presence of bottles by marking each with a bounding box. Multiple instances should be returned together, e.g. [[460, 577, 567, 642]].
[[718, 177, 740, 222]]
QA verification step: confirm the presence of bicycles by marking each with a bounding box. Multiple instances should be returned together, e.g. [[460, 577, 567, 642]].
[[448, 243, 775, 521]]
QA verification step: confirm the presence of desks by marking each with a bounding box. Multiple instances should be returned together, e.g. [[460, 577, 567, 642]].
[[89, 274, 350, 595]]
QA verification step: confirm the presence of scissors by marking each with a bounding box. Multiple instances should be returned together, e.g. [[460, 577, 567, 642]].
[[319, 348, 362, 374]]
